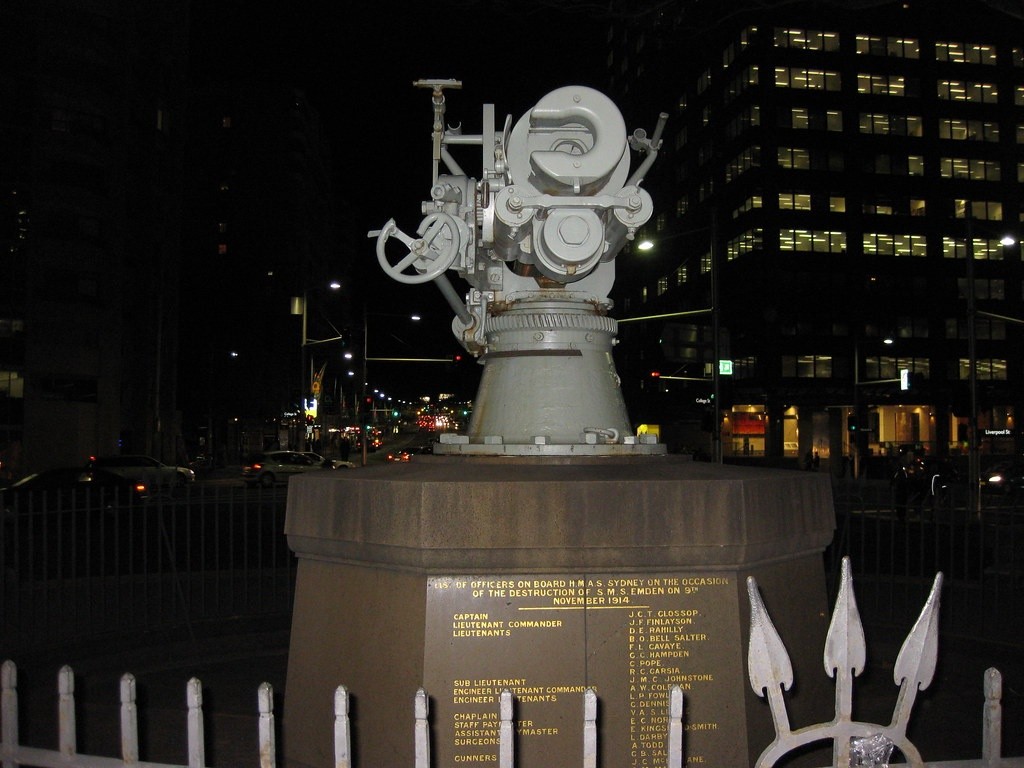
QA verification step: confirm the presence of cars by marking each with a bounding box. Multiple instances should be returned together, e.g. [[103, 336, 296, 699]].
[[0, 463, 142, 516], [888, 452, 969, 492], [76, 453, 197, 506], [976, 461, 1024, 496], [383, 446, 432, 465]]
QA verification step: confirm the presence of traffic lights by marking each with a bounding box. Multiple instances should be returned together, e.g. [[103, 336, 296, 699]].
[[848, 415, 856, 432]]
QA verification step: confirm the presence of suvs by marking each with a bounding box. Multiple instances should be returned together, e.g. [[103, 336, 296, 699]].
[[243, 450, 357, 489]]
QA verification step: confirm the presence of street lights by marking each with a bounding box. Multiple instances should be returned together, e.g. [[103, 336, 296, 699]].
[[964, 199, 1014, 491], [294, 282, 340, 459], [358, 312, 423, 467], [638, 206, 721, 465]]
